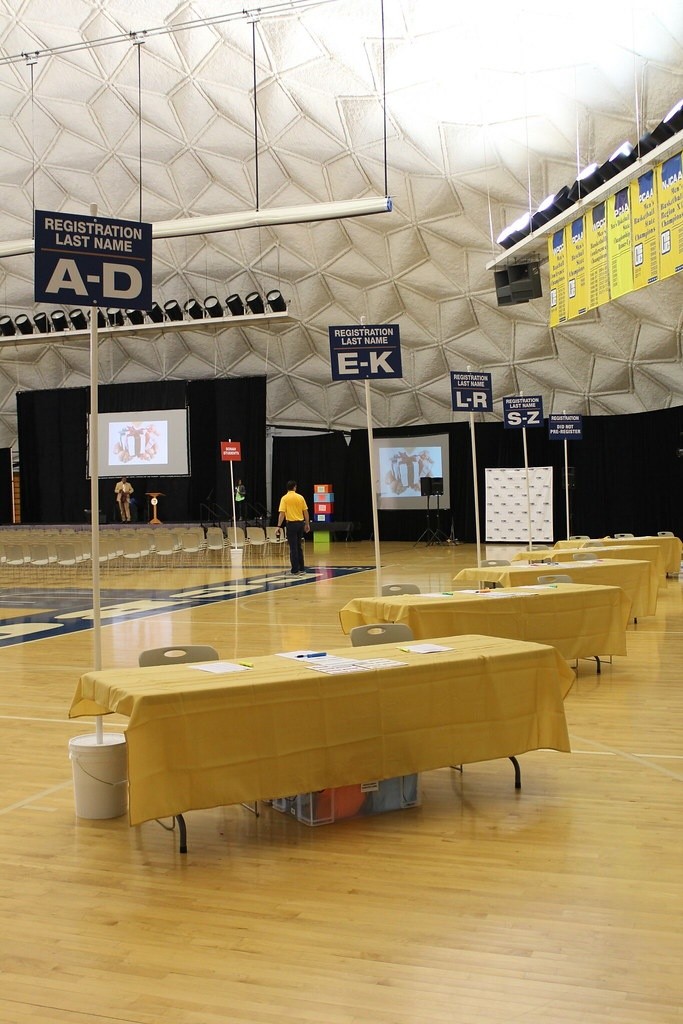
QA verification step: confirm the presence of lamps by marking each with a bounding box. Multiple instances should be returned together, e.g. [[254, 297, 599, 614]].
[[497, 99, 683, 249], [0, 287, 286, 338]]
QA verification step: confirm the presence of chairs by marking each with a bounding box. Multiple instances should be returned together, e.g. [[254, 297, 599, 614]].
[[526, 545, 551, 563], [0, 526, 288, 596], [139, 645, 219, 667], [481, 560, 511, 589], [582, 540, 604, 548], [614, 534, 634, 538], [382, 584, 420, 596], [537, 574, 572, 584], [573, 553, 598, 560], [350, 624, 414, 647], [657, 532, 674, 537], [569, 536, 590, 540]]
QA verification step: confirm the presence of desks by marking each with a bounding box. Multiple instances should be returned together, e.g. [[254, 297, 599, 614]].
[[554, 537, 683, 578], [82, 635, 557, 855], [464, 558, 651, 624], [353, 583, 623, 676], [304, 522, 352, 542], [520, 545, 661, 570]]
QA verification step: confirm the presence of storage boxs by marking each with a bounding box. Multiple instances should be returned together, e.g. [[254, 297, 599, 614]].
[[314, 493, 334, 502], [314, 503, 333, 515], [314, 484, 332, 493], [314, 515, 331, 523]]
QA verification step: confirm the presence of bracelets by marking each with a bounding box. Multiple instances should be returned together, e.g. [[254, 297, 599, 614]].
[[277, 526, 281, 527]]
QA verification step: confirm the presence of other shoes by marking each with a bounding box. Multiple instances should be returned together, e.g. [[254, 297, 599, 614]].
[[120, 521, 125, 524], [293, 572, 299, 578], [125, 521, 131, 524], [299, 570, 306, 575], [239, 516, 243, 521]]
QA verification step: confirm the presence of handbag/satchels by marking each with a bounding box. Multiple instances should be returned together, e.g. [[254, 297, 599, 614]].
[[121, 491, 128, 503]]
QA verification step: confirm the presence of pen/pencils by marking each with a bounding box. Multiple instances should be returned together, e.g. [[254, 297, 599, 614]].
[[397, 647, 408, 653], [442, 592, 453, 595], [549, 585, 558, 587], [475, 590, 491, 593], [296, 652, 327, 658], [239, 662, 253, 667]]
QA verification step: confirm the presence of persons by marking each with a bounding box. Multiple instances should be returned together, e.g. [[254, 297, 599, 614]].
[[275, 480, 310, 575], [114, 476, 133, 523], [233, 479, 246, 521]]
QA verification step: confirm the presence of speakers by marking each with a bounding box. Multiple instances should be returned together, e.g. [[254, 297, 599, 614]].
[[494, 262, 542, 307], [431, 478, 444, 496], [420, 476, 431, 496]]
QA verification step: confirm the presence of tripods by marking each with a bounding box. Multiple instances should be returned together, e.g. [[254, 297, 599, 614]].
[[413, 495, 458, 548]]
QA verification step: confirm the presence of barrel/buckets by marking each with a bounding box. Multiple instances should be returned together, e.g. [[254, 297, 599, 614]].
[[230, 548, 243, 565], [67, 732, 128, 820]]
